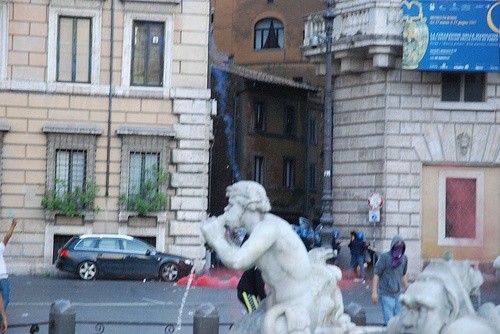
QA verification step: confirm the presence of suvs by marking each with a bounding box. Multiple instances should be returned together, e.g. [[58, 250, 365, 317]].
[[55, 234, 194, 283]]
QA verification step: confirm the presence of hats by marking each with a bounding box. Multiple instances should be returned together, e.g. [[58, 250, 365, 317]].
[[357, 232, 364, 239], [333, 230, 339, 239]]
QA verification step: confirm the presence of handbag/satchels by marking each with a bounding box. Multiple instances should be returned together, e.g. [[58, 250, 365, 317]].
[[364, 246, 371, 262]]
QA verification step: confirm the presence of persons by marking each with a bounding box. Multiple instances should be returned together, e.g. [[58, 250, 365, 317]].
[[0, 218, 18, 323], [384, 256, 500, 334], [200, 181, 355, 334], [372, 235, 409, 327], [290, 217, 371, 284], [0, 293, 8, 334]]
